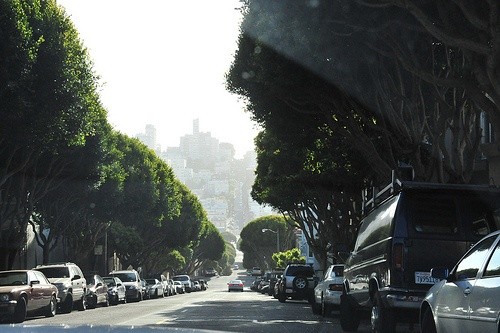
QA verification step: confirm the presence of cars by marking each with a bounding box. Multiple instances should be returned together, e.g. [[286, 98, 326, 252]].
[[312, 263, 346, 317], [102, 276, 127, 305], [418, 229, 500, 333], [249, 268, 285, 297], [251, 266, 262, 276], [227, 279, 244, 292], [0, 270, 59, 324], [140, 273, 209, 300], [83, 273, 110, 310]]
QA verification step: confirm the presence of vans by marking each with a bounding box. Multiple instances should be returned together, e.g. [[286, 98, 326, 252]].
[[108, 269, 143, 303]]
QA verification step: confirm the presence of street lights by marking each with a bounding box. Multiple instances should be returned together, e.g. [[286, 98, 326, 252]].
[[261, 228, 280, 267]]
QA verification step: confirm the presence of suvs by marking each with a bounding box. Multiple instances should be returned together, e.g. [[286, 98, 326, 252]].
[[30, 261, 88, 314], [338, 164, 500, 333], [277, 263, 319, 304]]
